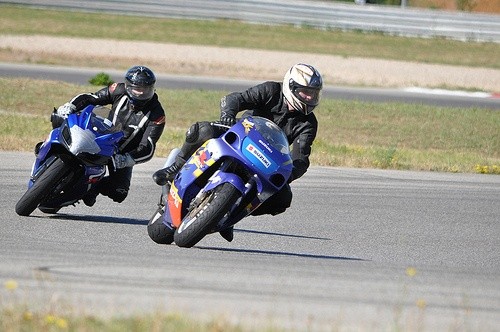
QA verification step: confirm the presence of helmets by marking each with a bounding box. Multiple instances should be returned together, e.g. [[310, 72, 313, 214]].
[[125, 66, 156, 104], [283, 63, 324, 116]]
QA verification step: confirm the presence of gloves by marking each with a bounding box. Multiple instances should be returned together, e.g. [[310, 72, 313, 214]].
[[115, 152, 135, 169], [220, 113, 237, 126], [56, 103, 77, 118]]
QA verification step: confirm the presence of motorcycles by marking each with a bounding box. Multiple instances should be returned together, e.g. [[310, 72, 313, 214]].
[[14, 105, 136, 217], [147, 109, 304, 249]]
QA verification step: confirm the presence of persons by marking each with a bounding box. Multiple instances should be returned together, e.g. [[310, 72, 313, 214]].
[[34, 66, 165, 207], [153, 62, 322, 242]]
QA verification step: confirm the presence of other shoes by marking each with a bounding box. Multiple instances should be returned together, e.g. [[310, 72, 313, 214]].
[[84, 189, 100, 206]]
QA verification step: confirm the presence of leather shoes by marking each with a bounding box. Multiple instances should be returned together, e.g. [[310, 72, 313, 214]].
[[153, 162, 182, 186], [220, 227, 233, 242]]
